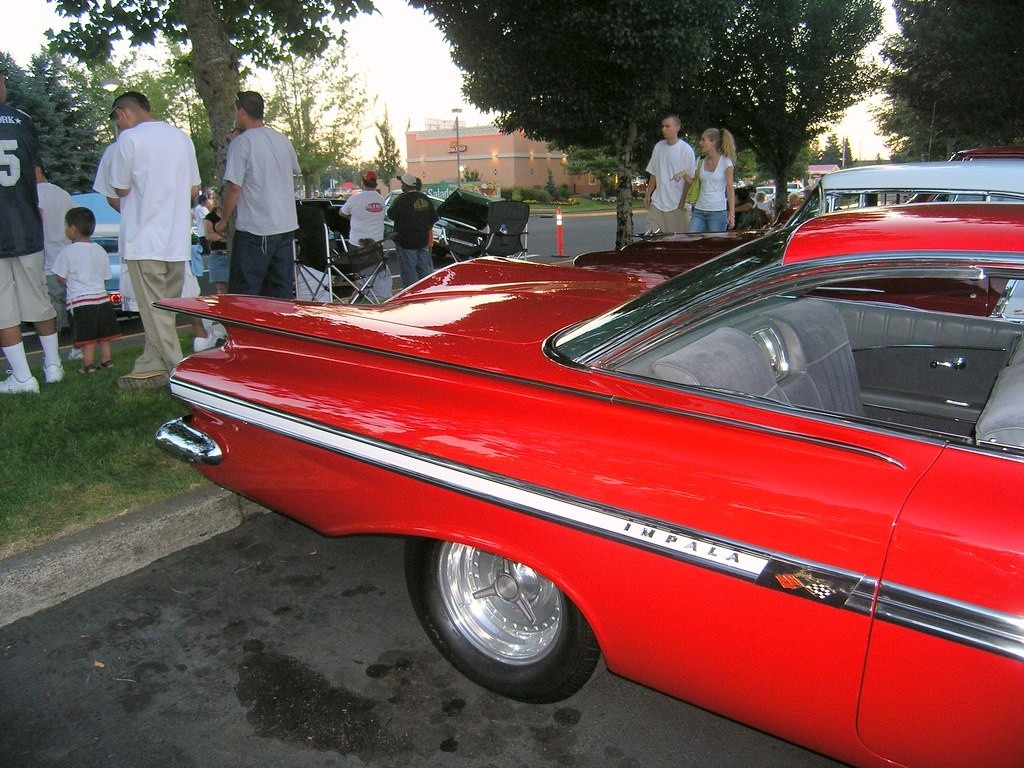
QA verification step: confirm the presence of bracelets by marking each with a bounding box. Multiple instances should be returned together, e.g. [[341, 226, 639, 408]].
[[213, 223, 216, 232], [730, 215, 735, 217]]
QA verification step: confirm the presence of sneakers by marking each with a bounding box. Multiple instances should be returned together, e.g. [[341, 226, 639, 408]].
[[194, 329, 226, 352], [0, 376, 39, 393], [44, 365, 65, 383]]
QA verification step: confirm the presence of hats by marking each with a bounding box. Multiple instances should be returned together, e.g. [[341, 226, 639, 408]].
[[198, 195, 207, 202], [363, 171, 377, 183], [397, 175, 416, 187], [34, 157, 52, 173]]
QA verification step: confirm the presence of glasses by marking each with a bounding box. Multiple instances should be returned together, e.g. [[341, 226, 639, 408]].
[[236, 91, 263, 115], [0, 69, 8, 75], [109, 107, 120, 121]]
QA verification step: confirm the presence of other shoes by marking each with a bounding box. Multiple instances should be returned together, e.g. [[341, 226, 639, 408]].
[[119, 371, 168, 387], [216, 339, 226, 347]]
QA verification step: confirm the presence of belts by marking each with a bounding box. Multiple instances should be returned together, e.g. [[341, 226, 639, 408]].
[[211, 252, 228, 256]]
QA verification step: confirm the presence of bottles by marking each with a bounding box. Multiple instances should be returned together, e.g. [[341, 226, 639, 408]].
[[500, 224, 508, 234]]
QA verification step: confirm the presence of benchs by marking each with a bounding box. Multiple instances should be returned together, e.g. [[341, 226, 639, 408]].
[[651, 301, 863, 418]]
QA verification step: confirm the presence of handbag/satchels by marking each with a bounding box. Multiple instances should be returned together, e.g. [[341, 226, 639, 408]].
[[685, 161, 703, 204]]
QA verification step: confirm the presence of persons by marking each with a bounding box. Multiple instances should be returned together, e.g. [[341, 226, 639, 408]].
[[644, 114, 695, 232], [49, 207, 114, 373], [92, 91, 201, 389], [191, 189, 228, 295], [35, 158, 84, 359], [339, 172, 385, 250], [210, 91, 301, 300], [313, 190, 319, 199], [670, 128, 737, 232], [0, 69, 64, 393], [348, 238, 392, 304], [726, 185, 798, 230], [388, 173, 439, 290]]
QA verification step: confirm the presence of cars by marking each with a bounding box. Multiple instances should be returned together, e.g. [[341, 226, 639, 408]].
[[69, 192, 144, 320], [295, 185, 362, 198], [152, 202, 1024, 768], [573, 147, 1024, 322]]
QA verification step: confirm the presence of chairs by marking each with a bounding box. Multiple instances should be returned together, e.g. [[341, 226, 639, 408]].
[[435, 198, 530, 263], [975, 362, 1024, 449], [292, 205, 398, 304]]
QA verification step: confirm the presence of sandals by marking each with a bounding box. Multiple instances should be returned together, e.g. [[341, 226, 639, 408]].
[[97, 361, 116, 368], [76, 364, 97, 374]]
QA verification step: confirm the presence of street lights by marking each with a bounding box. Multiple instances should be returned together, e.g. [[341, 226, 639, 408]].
[[451, 109, 463, 188], [100, 77, 121, 142]]
